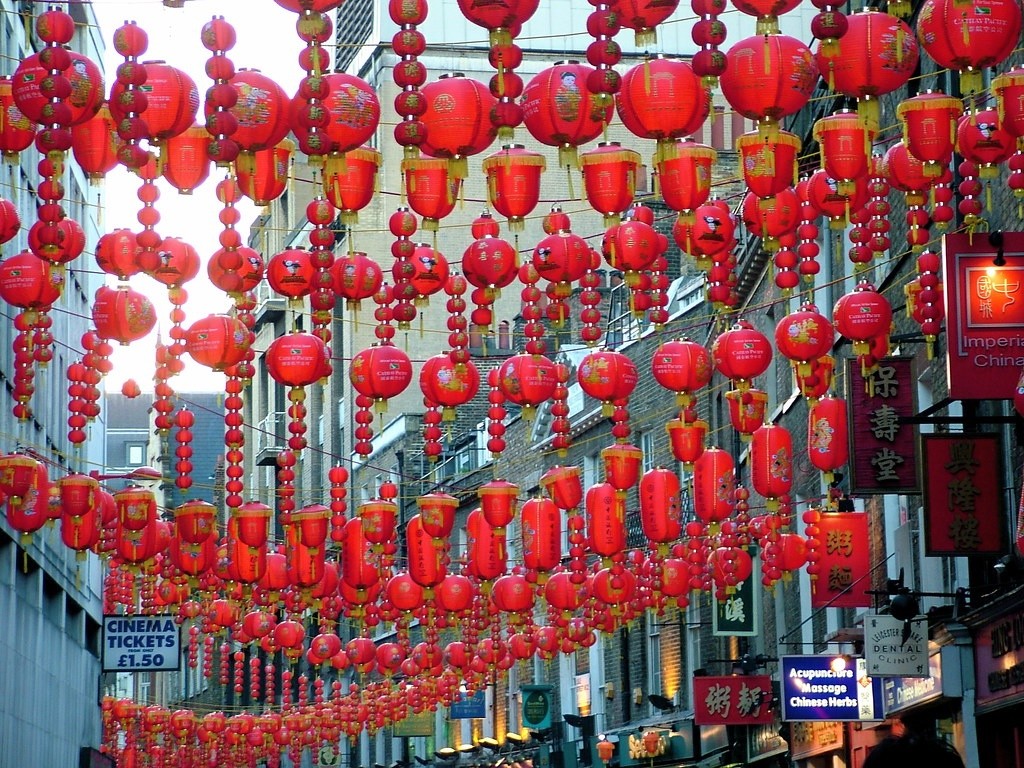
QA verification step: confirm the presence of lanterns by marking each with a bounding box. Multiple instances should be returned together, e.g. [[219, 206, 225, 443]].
[[1, 0, 1024, 768]]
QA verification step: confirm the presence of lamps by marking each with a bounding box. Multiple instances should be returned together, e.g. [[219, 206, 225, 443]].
[[648, 695, 674, 710], [396, 760, 413, 767], [507, 737, 525, 746], [951, 587, 990, 620], [563, 715, 586, 727], [478, 742, 499, 751], [374, 763, 385, 768], [459, 747, 479, 753], [994, 562, 1024, 586], [414, 756, 432, 766], [434, 751, 458, 760], [529, 733, 544, 741], [989, 231, 1006, 268]]
